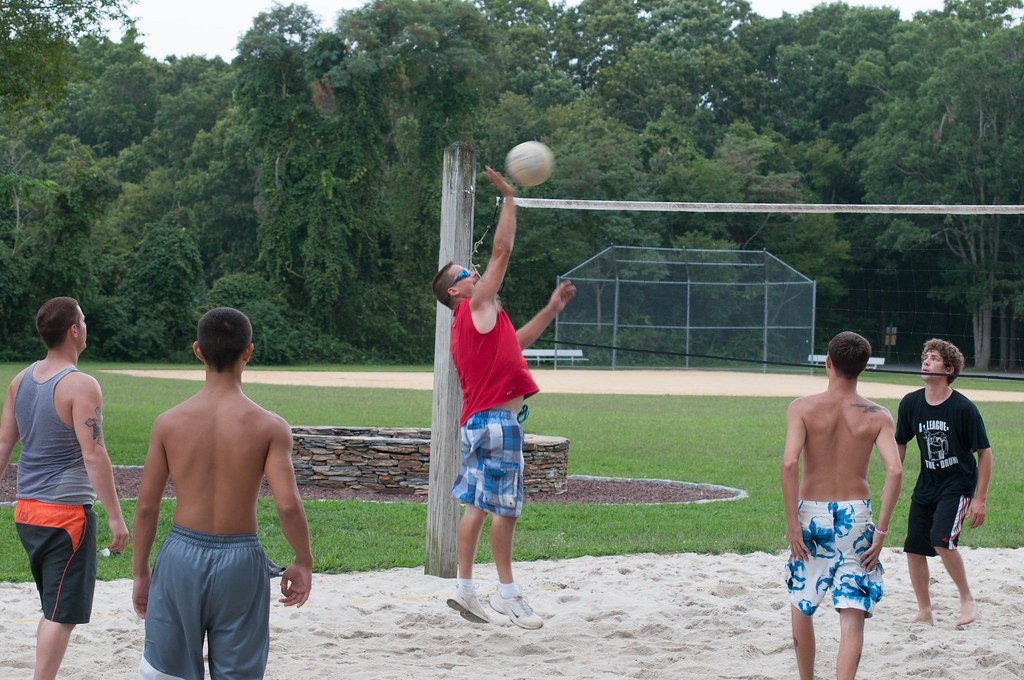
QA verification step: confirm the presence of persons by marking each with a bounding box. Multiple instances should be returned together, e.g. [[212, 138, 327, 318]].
[[781, 330, 904, 680], [880, 337, 992, 626], [431, 164, 575, 629], [132, 306, 312, 680], [0, 296, 129, 680]]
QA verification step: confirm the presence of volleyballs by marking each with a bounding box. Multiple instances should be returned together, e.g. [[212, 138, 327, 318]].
[[502, 141, 558, 187]]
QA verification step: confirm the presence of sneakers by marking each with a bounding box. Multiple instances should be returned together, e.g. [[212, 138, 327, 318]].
[[489, 578, 544, 630], [447, 582, 490, 624]]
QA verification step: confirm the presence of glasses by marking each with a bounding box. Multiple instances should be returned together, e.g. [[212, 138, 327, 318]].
[[447, 268, 470, 297]]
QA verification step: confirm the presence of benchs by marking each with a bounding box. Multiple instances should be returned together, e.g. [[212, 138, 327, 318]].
[[808, 353, 885, 370], [520, 349, 589, 367]]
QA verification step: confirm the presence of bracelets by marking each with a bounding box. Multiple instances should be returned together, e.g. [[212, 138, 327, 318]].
[[875, 526, 889, 535]]
